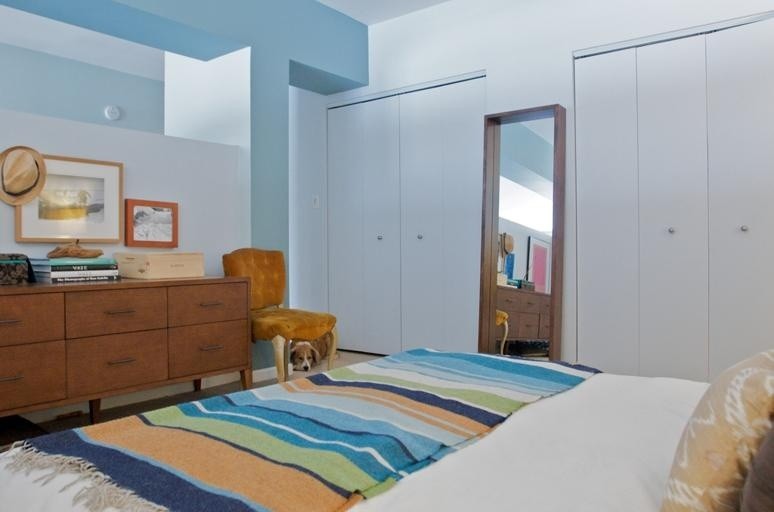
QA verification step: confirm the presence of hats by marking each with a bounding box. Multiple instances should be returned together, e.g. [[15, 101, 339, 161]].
[[0, 146, 47, 206], [501, 233, 513, 258]]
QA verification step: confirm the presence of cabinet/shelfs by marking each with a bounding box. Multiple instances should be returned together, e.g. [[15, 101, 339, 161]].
[[1, 276, 66, 419], [319, 66, 482, 363], [494, 284, 552, 344], [565, 7, 772, 382], [60, 281, 168, 423], [163, 273, 253, 397]]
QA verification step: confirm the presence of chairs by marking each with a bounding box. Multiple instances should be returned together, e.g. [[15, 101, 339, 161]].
[[494, 307, 512, 356], [219, 246, 339, 385]]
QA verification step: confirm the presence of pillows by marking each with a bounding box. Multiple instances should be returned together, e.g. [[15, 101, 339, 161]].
[[657, 347, 773, 510], [739, 429, 773, 511]]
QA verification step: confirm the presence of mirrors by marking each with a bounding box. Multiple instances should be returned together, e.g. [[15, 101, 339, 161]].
[[475, 100, 568, 365]]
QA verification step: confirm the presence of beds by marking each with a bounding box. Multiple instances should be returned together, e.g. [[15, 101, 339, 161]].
[[1, 343, 774, 510]]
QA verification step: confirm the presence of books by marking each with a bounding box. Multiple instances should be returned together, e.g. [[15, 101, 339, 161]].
[[49, 254, 119, 284]]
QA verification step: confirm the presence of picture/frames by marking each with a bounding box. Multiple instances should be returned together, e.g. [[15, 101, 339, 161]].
[[122, 193, 178, 248], [12, 149, 125, 246], [524, 235, 552, 296]]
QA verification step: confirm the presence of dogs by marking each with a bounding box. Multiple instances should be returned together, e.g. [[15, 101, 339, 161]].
[[288, 333, 333, 371]]
